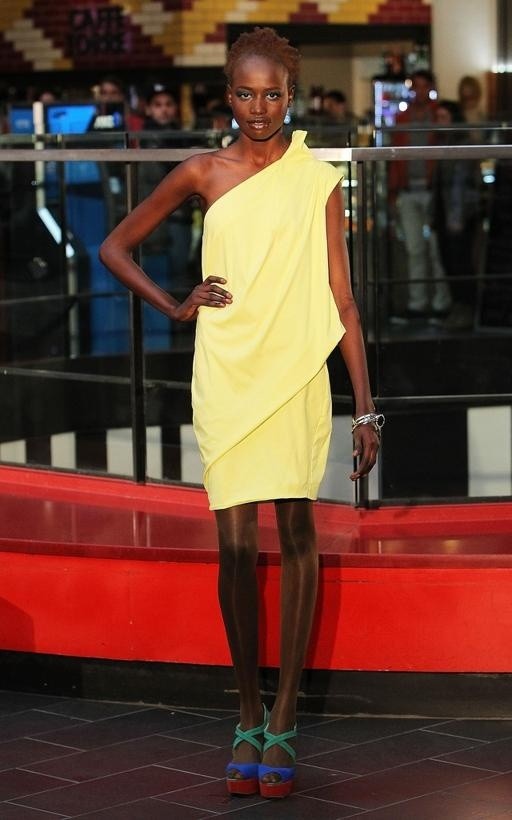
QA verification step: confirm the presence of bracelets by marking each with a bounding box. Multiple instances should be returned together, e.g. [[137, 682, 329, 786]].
[[351, 410, 388, 440]]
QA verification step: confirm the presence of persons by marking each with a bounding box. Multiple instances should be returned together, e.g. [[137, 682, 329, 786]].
[[4, 52, 492, 329], [94, 26, 390, 797]]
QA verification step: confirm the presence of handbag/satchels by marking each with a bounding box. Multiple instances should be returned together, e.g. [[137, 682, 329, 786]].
[[351, 410, 388, 440]]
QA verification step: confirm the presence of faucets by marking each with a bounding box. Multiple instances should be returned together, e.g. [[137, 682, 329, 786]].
[[258, 712, 301, 797], [226, 703, 257, 796]]
